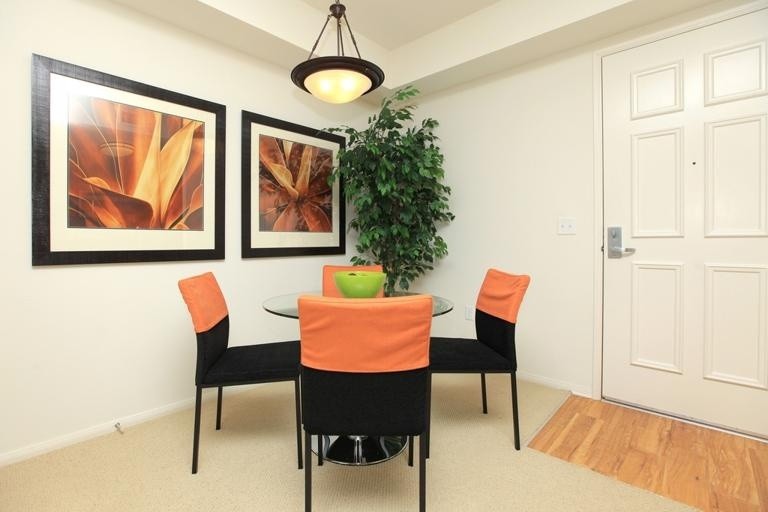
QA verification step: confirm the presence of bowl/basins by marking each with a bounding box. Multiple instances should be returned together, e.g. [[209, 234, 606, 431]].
[[334, 270, 388, 300]]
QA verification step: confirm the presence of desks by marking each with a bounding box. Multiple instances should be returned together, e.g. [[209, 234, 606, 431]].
[[264, 290, 452, 468]]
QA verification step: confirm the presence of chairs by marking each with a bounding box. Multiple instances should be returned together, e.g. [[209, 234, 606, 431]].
[[321, 267, 387, 296], [295, 294, 433, 511], [424, 269, 528, 458], [178, 272, 303, 474]]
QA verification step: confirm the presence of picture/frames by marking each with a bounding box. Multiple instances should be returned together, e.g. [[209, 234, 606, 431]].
[[241, 111, 345, 256], [31, 52, 228, 265]]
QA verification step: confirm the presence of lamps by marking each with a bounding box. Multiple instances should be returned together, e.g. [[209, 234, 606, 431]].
[[290, 0, 385, 106]]
